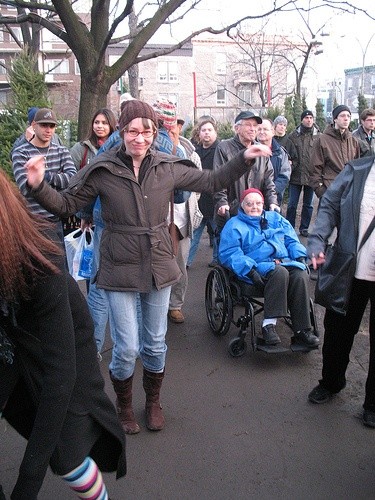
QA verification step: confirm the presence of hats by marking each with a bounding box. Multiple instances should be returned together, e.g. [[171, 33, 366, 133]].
[[27, 105, 39, 125], [150, 96, 178, 128], [240, 188, 266, 203], [301, 110, 313, 120], [117, 91, 158, 130], [234, 110, 262, 125], [34, 108, 59, 127], [332, 105, 351, 119]]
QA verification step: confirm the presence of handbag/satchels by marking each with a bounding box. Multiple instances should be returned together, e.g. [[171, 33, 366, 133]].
[[313, 246, 356, 317], [63, 227, 98, 282]]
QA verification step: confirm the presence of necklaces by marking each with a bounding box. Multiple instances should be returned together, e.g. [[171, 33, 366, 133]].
[[133, 166, 140, 168], [31, 139, 51, 168]]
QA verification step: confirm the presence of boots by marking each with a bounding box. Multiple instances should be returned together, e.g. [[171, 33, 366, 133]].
[[108, 369, 141, 434], [142, 364, 167, 431]]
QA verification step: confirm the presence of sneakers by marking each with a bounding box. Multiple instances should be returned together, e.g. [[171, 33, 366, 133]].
[[308, 376, 348, 404], [363, 403, 375, 429]]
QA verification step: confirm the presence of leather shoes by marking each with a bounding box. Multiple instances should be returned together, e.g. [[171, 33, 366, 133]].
[[295, 330, 322, 348], [261, 323, 282, 345]]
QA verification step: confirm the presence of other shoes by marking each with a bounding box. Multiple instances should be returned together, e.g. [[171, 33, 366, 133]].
[[168, 308, 185, 323]]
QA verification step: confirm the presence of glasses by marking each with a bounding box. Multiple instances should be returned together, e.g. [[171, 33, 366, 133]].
[[122, 128, 156, 139], [238, 122, 259, 128], [365, 119, 375, 122]]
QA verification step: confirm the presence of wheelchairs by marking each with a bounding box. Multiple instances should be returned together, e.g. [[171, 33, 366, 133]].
[[204, 209, 320, 356]]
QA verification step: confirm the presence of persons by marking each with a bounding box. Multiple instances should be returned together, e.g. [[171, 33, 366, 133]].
[[303, 145, 375, 429], [254, 118, 292, 215], [349, 108, 375, 163], [150, 96, 226, 324], [309, 105, 361, 220], [12, 108, 80, 268], [213, 111, 283, 307], [70, 109, 120, 297], [0, 169, 129, 500], [282, 110, 325, 238], [272, 114, 296, 171], [218, 187, 322, 349], [69, 92, 190, 368], [9, 106, 64, 161], [26, 99, 272, 435]]
